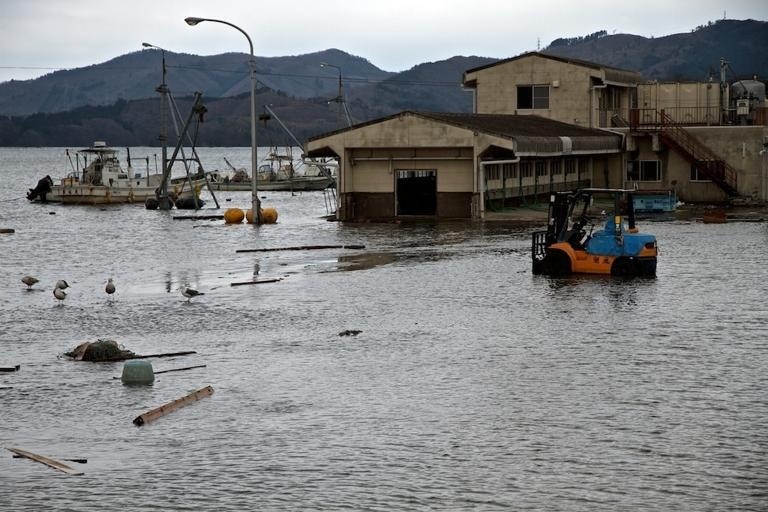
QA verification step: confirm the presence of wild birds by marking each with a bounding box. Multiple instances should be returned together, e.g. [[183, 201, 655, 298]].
[[52, 285, 68, 305], [176, 285, 205, 302], [56, 278, 69, 291], [105, 277, 115, 295], [21, 276, 41, 289]]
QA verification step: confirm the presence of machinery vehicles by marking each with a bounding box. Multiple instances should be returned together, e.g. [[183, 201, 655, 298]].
[[523, 182, 661, 285]]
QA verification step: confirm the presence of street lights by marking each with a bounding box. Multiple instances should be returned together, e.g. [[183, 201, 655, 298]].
[[181, 15, 264, 228], [140, 40, 168, 200], [319, 59, 345, 132]]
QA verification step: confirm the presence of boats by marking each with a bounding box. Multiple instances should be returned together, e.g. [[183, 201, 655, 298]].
[[24, 137, 209, 207], [202, 147, 335, 194]]
